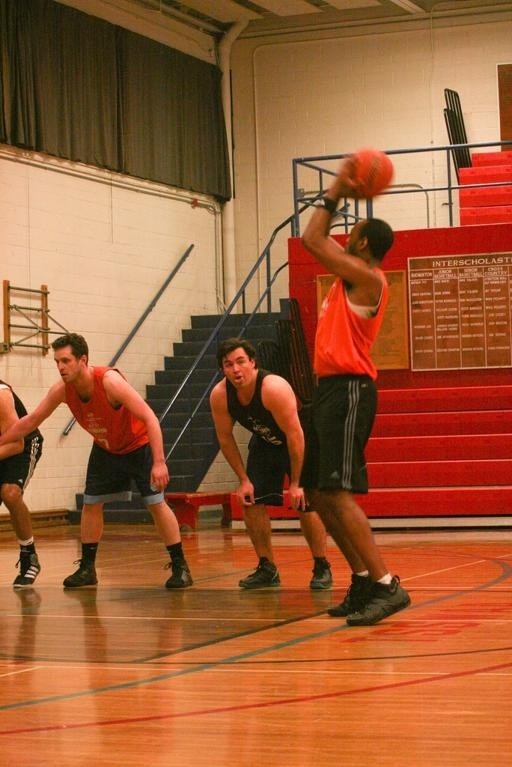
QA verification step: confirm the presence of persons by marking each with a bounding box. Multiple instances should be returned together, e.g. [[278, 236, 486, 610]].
[[300, 154, 411, 626], [210, 338, 332, 590], [0, 334, 193, 590], [0, 380, 44, 588]]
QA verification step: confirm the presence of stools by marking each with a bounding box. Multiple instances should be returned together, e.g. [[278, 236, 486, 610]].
[[163, 491, 233, 532]]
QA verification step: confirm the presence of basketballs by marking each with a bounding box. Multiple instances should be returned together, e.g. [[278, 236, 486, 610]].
[[350, 149, 392, 196]]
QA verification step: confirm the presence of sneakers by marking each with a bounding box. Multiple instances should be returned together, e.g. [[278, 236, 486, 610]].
[[165, 562, 193, 588], [63, 559, 98, 587], [13, 552, 41, 588], [310, 560, 332, 589], [327, 574, 411, 627], [239, 559, 280, 589]]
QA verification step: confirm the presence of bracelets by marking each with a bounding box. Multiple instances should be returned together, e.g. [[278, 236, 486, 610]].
[[314, 197, 336, 213]]
